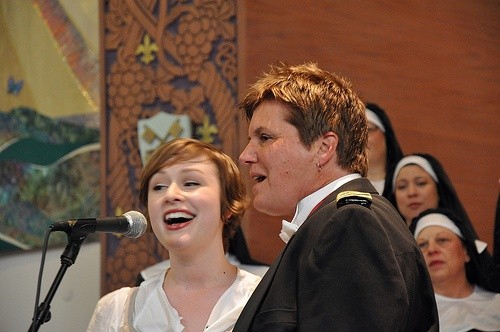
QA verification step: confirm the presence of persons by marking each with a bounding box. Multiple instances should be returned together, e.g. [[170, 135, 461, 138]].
[[362, 102, 406, 222], [85, 136, 261, 332], [408, 208, 500, 332], [233, 60, 439, 332], [389, 152, 494, 290], [136, 227, 270, 288]]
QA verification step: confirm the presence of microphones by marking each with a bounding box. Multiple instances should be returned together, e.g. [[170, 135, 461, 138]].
[[49, 211, 147, 239]]
